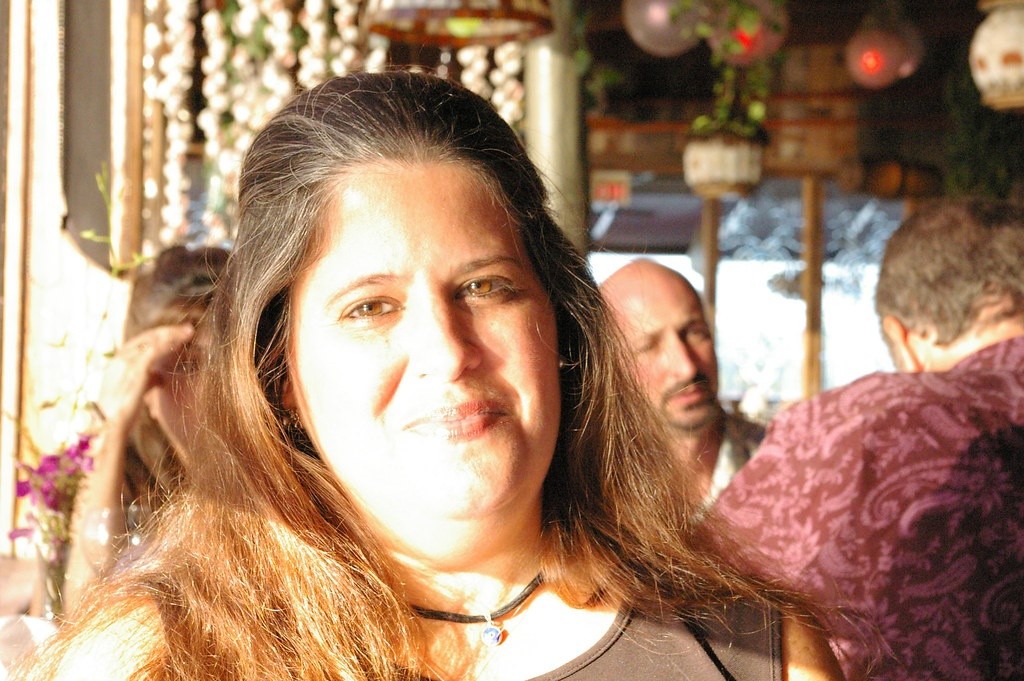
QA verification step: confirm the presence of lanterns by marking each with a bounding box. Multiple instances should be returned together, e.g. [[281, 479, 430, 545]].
[[846, 18, 924, 88], [703, 0, 788, 65], [623, 0, 717, 58], [968, 0, 1024, 107]]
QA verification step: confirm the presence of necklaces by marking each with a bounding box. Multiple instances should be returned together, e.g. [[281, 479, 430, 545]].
[[409, 571, 542, 646]]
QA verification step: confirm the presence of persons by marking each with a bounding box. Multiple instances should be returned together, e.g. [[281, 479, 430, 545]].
[[41, 72, 847, 680], [25, 245, 232, 630], [694, 194, 1024, 680], [600, 258, 764, 510]]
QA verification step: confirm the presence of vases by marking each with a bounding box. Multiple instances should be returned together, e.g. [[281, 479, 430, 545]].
[[31, 543, 72, 620]]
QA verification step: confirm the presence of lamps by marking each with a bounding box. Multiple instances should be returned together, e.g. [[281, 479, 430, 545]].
[[968, 0, 1024, 114], [845, 0, 925, 89], [680, 115, 766, 199], [619, 0, 790, 64]]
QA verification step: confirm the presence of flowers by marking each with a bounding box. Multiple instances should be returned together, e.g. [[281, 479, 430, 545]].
[[7, 417, 103, 569]]
